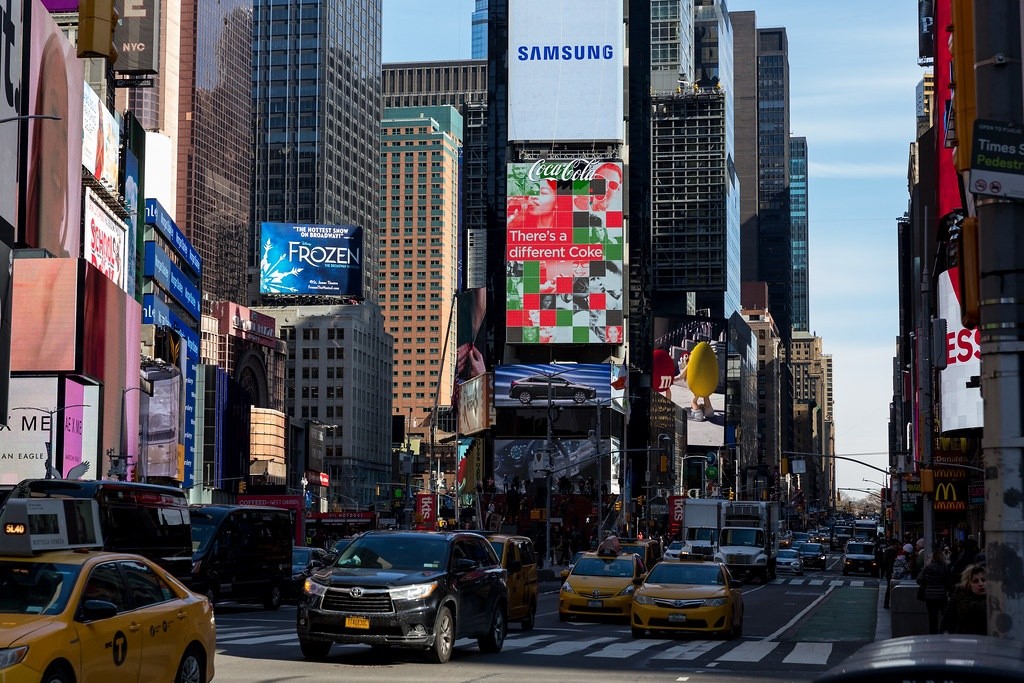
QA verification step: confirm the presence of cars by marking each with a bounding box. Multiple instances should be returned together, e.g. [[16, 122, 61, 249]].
[[510, 374, 596, 404], [631, 553, 743, 641], [775, 512, 885, 578], [0, 496, 216, 683], [666, 541, 682, 560], [561, 552, 584, 584], [291, 546, 327, 593], [558, 538, 650, 621]]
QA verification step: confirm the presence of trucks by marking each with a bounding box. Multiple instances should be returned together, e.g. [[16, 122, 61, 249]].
[[680, 497, 779, 582], [852, 520, 877, 539], [830, 521, 852, 550]]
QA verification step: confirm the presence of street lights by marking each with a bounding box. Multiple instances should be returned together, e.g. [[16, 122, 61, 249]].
[[717, 440, 748, 486], [12, 405, 87, 479], [546, 365, 601, 569], [577, 396, 640, 545], [680, 455, 708, 495], [657, 433, 671, 490]]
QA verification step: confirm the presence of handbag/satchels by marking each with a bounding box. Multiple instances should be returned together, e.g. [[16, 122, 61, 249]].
[[917, 585, 928, 601]]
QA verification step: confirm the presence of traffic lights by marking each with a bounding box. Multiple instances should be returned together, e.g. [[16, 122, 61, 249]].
[[729, 489, 734, 501], [450, 519, 455, 524], [637, 496, 642, 505], [616, 501, 620, 511], [242, 482, 246, 489], [439, 520, 447, 527]]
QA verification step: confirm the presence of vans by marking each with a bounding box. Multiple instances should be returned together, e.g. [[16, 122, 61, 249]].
[[189, 502, 294, 610], [484, 533, 539, 631], [618, 539, 662, 571]]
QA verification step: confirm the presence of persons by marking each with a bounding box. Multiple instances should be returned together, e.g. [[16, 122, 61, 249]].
[[503, 473, 531, 493], [916, 552, 948, 634], [457, 288, 487, 382], [567, 505, 578, 528], [601, 481, 609, 496], [883, 539, 898, 609], [507, 163, 623, 343], [941, 539, 978, 581], [976, 548, 986, 562], [891, 543, 913, 580], [476, 476, 496, 501], [558, 474, 594, 495], [505, 484, 520, 526], [914, 537, 924, 579], [534, 526, 590, 566], [521, 496, 529, 517], [944, 563, 987, 636], [636, 531, 665, 541], [602, 493, 616, 521]]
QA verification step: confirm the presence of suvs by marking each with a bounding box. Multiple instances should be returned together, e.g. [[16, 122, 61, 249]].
[[298, 529, 509, 664]]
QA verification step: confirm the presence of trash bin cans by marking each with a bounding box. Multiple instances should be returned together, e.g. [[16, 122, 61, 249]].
[[889, 585, 930, 638], [553, 551, 563, 564]]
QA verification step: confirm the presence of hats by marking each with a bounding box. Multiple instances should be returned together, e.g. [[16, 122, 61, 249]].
[[903, 543, 913, 552], [916, 538, 924, 546]]
